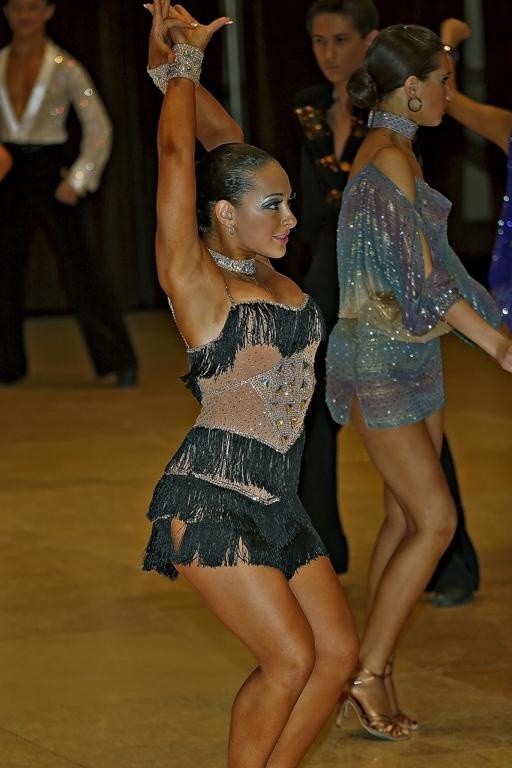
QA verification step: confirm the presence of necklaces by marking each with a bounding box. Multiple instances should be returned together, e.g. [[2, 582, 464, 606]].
[[367, 109, 419, 141], [206, 248, 256, 275]]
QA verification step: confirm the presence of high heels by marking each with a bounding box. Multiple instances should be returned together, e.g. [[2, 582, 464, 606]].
[[344, 661, 418, 735], [334, 667, 412, 742]]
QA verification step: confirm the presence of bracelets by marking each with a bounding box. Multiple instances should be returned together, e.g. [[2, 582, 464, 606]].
[[442, 44, 455, 57], [167, 43, 204, 86], [146, 63, 168, 96]]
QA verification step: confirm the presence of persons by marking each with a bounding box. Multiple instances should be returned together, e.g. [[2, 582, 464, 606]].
[[0, 0, 137, 385], [325, 25, 512, 741], [142, 0, 360, 768], [270, 0, 479, 608], [439, 18, 512, 344]]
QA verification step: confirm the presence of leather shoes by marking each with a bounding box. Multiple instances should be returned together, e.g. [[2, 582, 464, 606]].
[[428, 587, 476, 608], [329, 544, 349, 575]]
